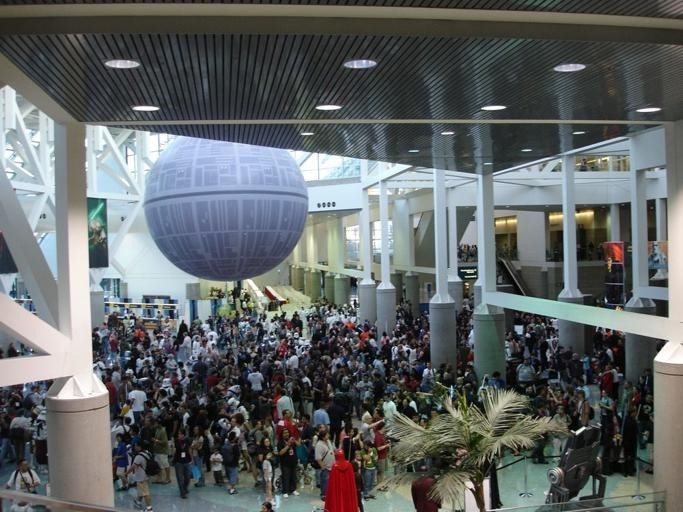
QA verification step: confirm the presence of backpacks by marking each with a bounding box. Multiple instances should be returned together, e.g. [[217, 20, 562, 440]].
[[137, 450, 160, 476], [223, 443, 236, 463], [122, 426, 132, 444]]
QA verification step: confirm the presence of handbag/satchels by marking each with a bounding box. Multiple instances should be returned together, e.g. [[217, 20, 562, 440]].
[[247, 441, 257, 455], [311, 460, 321, 470], [168, 442, 177, 465]]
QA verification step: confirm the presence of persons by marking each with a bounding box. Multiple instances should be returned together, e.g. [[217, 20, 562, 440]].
[[0, 235, 653, 512]]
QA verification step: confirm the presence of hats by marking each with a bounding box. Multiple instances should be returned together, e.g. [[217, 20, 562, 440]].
[[163, 378, 171, 388]]
